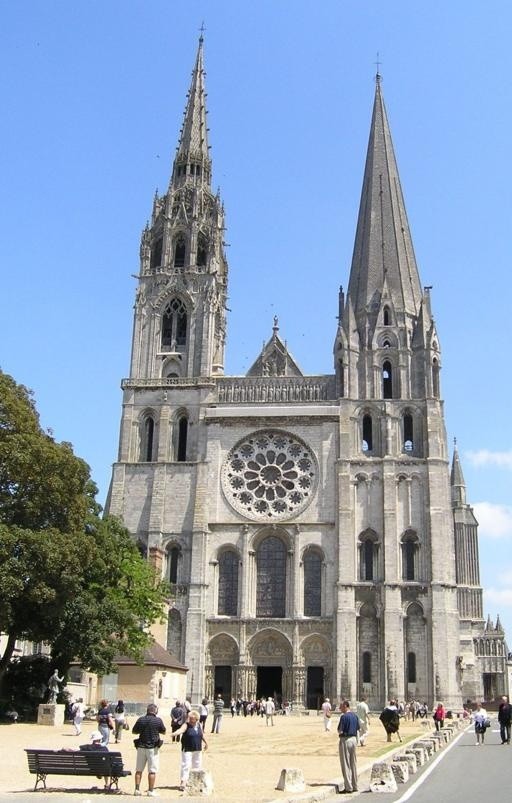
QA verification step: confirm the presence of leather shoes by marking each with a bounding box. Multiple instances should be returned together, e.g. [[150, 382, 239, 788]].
[[339, 789, 358, 794]]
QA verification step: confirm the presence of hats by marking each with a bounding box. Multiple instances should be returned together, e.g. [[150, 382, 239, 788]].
[[90, 731, 103, 741]]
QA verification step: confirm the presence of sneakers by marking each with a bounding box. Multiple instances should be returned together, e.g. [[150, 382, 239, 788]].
[[134, 789, 141, 796], [147, 791, 155, 796]]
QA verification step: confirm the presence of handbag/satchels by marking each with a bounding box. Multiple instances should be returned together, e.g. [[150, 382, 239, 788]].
[[123, 724, 129, 730]]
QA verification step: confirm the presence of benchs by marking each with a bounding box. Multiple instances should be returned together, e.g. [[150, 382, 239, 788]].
[[22, 747, 133, 793]]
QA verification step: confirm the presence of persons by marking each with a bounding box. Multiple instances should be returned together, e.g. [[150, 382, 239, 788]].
[[71, 696, 87, 735], [462, 706, 470, 718], [170, 708, 209, 791], [130, 701, 168, 797], [45, 668, 64, 703], [171, 686, 279, 743], [96, 698, 115, 745], [474, 701, 492, 746], [113, 699, 125, 743], [320, 693, 446, 742], [335, 699, 363, 793], [87, 729, 113, 790], [497, 695, 512, 744]]
